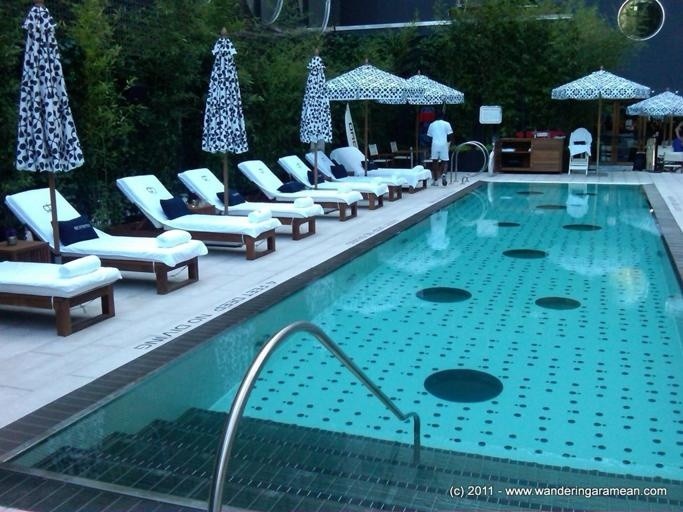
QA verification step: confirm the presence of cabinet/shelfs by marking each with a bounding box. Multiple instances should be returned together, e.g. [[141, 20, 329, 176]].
[[492, 135, 567, 175]]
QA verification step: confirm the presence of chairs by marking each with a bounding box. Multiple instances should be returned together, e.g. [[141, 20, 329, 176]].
[[565, 183, 589, 220], [567, 127, 593, 176], [0, 145, 442, 338]]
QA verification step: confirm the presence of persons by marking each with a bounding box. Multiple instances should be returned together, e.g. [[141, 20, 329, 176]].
[[426, 209, 451, 257], [426, 110, 454, 186]]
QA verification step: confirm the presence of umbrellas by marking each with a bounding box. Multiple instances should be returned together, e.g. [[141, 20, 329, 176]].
[[624, 86, 682, 148], [16, 5, 86, 268], [551, 64, 652, 173], [199, 26, 247, 213], [374, 67, 466, 168], [302, 54, 333, 187], [326, 54, 424, 180]]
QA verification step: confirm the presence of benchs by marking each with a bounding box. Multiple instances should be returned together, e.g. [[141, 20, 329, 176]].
[[655, 151, 682, 174]]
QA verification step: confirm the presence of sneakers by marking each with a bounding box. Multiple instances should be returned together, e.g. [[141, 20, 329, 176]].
[[442, 173, 447, 186], [431, 180, 438, 186]]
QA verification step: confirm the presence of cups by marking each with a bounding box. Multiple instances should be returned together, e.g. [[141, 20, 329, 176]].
[[192, 200, 199, 208], [7, 235, 17, 246]]
